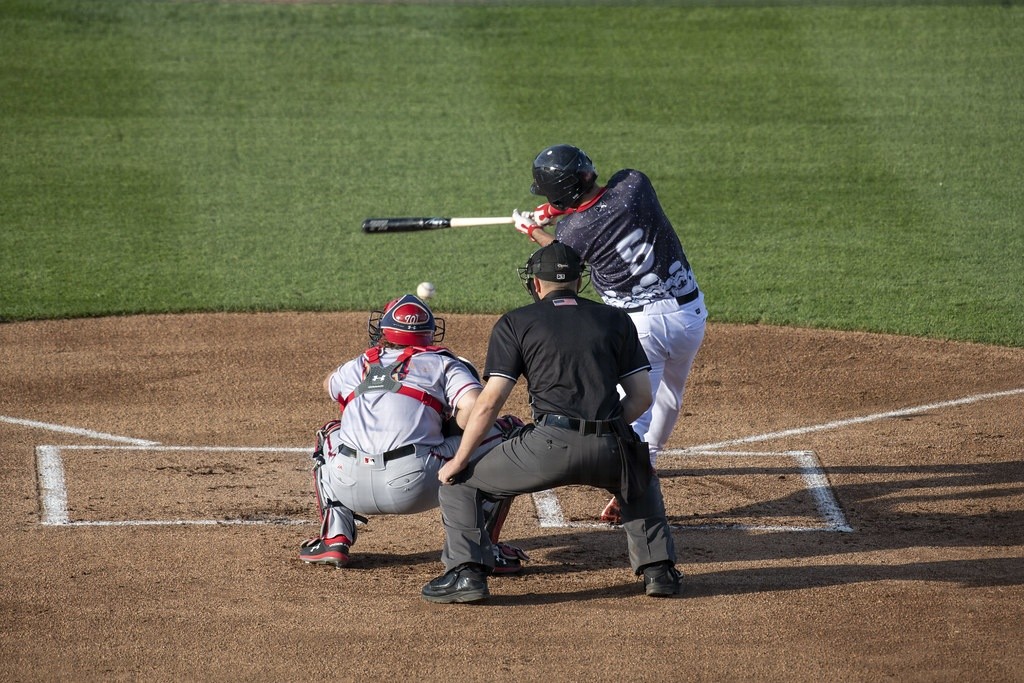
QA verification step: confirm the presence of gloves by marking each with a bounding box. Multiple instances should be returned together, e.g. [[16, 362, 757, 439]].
[[534, 203, 552, 226], [512, 206, 543, 242]]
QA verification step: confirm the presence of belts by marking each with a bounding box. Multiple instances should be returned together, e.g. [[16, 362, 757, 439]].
[[619, 287, 699, 313], [545, 415, 624, 434], [338, 444, 413, 462]]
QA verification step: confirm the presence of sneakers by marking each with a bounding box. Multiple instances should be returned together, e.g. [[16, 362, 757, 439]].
[[301, 538, 348, 566], [497, 551, 523, 575], [599, 496, 623, 526], [421, 568, 488, 603], [645, 566, 684, 595]]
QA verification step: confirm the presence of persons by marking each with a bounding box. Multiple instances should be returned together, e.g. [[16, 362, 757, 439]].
[[423, 239, 685, 604], [512, 144, 709, 521], [297, 292, 528, 574]]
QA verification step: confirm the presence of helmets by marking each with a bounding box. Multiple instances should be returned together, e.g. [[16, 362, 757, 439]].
[[369, 294, 446, 347], [517, 239, 593, 302]]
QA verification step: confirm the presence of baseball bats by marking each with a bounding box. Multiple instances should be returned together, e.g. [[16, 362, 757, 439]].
[[360, 216, 514, 233]]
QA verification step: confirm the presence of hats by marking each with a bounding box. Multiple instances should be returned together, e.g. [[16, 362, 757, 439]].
[[530, 145, 599, 211]]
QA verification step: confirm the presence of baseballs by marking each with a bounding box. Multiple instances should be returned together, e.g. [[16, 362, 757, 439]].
[[417, 282, 436, 300]]
